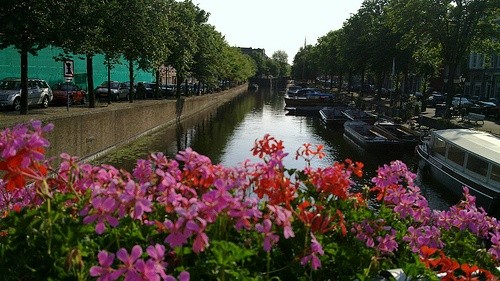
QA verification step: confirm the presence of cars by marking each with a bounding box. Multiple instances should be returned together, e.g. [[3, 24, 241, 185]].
[[318, 75, 332, 85], [50, 83, 86, 105], [374, 88, 389, 98], [411, 90, 498, 119], [94, 80, 236, 102]]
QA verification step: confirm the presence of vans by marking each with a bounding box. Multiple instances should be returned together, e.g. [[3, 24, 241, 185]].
[[0, 77, 54, 112]]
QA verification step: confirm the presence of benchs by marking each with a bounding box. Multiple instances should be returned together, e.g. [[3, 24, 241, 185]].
[[462, 112, 485, 126]]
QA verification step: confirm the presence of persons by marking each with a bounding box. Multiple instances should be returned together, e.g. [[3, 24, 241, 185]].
[[194, 83, 197, 91]]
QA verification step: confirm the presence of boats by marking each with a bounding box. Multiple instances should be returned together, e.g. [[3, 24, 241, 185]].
[[414, 128, 500, 219], [284, 85, 422, 153]]
